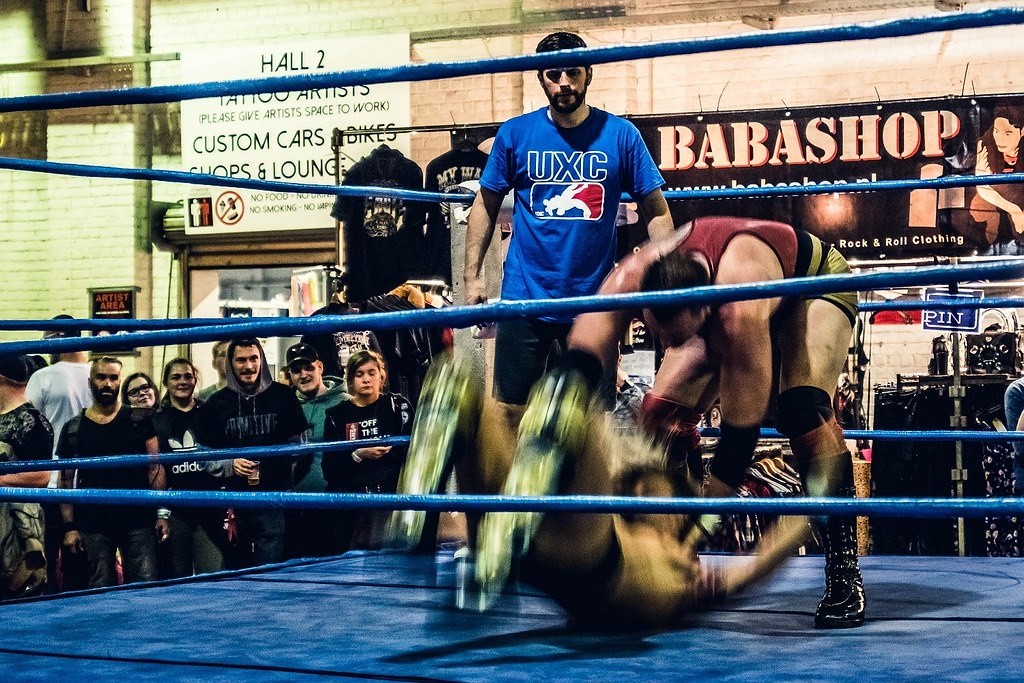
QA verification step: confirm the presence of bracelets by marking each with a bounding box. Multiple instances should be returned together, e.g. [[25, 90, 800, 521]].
[[62, 521, 79, 534], [156, 508, 173, 520]]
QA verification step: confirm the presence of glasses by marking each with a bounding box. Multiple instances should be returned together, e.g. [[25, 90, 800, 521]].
[[127, 384, 152, 397]]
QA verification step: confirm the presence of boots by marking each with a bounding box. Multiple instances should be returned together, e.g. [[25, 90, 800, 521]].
[[801, 487, 867, 629]]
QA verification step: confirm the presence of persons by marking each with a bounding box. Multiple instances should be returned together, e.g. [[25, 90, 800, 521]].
[[0, 351, 54, 600], [1004, 371, 1024, 557], [464, 31, 676, 463], [320, 352, 415, 553], [151, 357, 225, 580], [368, 346, 844, 645], [53, 355, 173, 591], [561, 214, 869, 628], [121, 370, 160, 412], [278, 342, 362, 562], [190, 335, 231, 405], [24, 314, 101, 593], [193, 334, 316, 575]]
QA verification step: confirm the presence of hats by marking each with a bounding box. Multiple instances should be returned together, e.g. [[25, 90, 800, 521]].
[[0, 351, 34, 385], [286, 342, 319, 369]]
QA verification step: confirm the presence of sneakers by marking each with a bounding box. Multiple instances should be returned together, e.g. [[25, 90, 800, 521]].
[[452, 546, 475, 563]]
